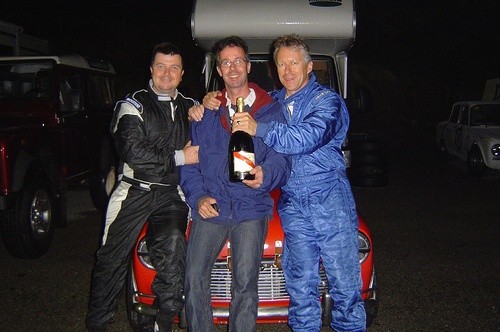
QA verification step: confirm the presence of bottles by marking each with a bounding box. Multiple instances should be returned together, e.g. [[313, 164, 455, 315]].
[[228, 97, 255, 181]]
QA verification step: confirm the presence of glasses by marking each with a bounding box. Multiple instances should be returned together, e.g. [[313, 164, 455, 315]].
[[216, 58, 249, 67]]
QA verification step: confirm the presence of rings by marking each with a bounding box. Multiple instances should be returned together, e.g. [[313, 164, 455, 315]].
[[237, 121, 240, 125]]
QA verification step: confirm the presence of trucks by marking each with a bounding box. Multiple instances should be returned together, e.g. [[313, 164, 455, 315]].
[[190, 0, 358, 181]]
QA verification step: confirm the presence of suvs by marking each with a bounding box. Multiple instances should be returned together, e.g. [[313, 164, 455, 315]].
[[439, 100, 500, 178], [0, 54, 129, 259]]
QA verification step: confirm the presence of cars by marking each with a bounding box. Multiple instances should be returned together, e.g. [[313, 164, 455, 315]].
[[96, 140, 381, 331]]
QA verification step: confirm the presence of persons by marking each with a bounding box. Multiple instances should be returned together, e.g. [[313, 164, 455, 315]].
[[86, 42, 205, 332], [177, 37, 292, 332], [231, 33, 367, 332]]
